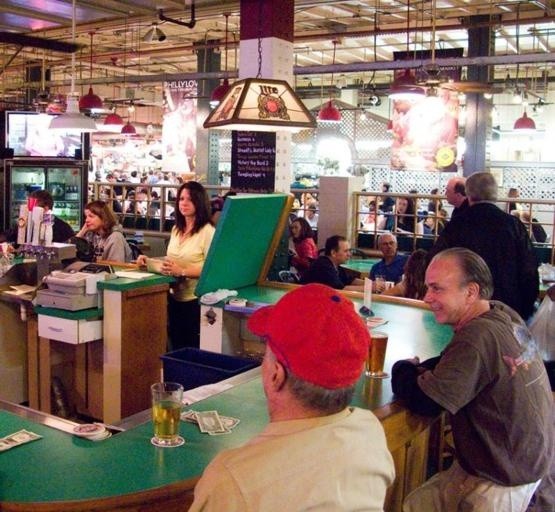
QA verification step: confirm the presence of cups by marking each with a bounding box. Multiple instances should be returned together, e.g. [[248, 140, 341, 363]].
[[366, 331, 389, 378], [150, 382, 184, 448], [375, 275, 385, 290]]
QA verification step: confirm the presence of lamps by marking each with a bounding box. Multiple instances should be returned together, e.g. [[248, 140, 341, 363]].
[[80, 33, 104, 112], [104, 57, 122, 125], [121, 109, 137, 134], [514, 106, 537, 132], [203, 0, 318, 132], [317, 40, 341, 123], [209, 11, 233, 104]]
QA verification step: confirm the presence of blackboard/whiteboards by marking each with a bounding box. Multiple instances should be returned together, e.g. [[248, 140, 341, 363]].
[[232, 129, 277, 196]]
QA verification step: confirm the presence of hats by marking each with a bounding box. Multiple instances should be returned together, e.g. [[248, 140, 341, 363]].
[[247, 283, 373, 390]]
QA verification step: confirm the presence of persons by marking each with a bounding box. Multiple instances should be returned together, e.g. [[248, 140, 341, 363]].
[[136, 181, 217, 353], [429, 172, 539, 320], [64, 201, 134, 262], [209, 192, 238, 227], [87, 171, 183, 219], [187, 282, 397, 512], [443, 177, 468, 222], [392, 248, 555, 512], [290, 185, 546, 300], [0, 189, 75, 257]]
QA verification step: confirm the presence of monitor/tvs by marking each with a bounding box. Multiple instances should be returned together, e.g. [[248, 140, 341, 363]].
[[4, 109, 91, 161]]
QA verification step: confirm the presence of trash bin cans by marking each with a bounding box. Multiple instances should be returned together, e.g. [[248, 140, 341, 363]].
[[159, 347, 261, 396]]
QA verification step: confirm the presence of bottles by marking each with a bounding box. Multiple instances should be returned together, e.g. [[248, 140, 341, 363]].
[[17, 245, 58, 274], [89, 237, 104, 262], [11, 182, 80, 225], [51, 376, 72, 419]]
